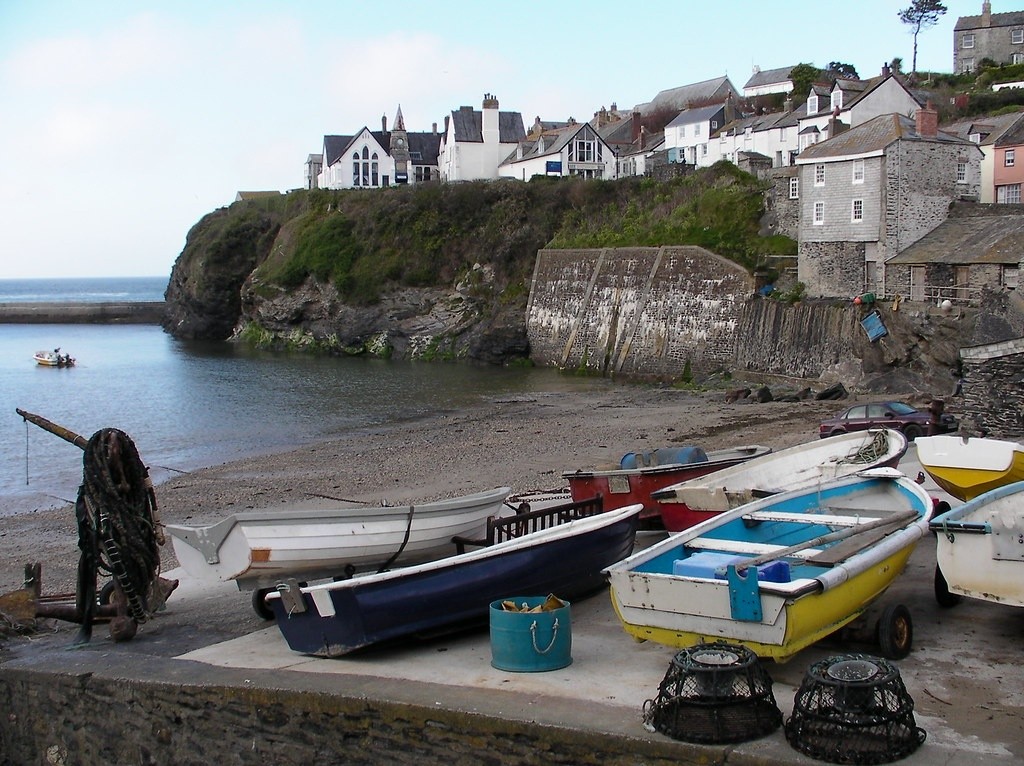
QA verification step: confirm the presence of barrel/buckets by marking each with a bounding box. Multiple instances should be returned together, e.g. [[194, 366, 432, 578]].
[[619, 448, 710, 470], [489, 597, 574, 673]]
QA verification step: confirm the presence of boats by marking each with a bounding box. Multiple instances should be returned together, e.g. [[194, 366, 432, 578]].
[[648, 425, 909, 539], [561, 442, 773, 527], [261, 500, 647, 660], [33, 350, 75, 367], [162, 483, 513, 594], [927, 479, 1024, 610], [589, 469, 933, 665], [914, 435, 1024, 503]]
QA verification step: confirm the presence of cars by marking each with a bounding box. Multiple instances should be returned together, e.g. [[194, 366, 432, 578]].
[[818, 400, 946, 441]]
